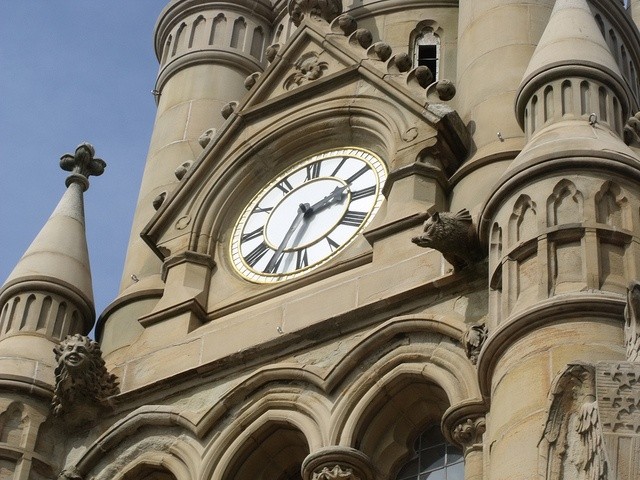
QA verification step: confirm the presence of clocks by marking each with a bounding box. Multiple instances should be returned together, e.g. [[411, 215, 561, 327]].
[[228, 146, 387, 285]]
